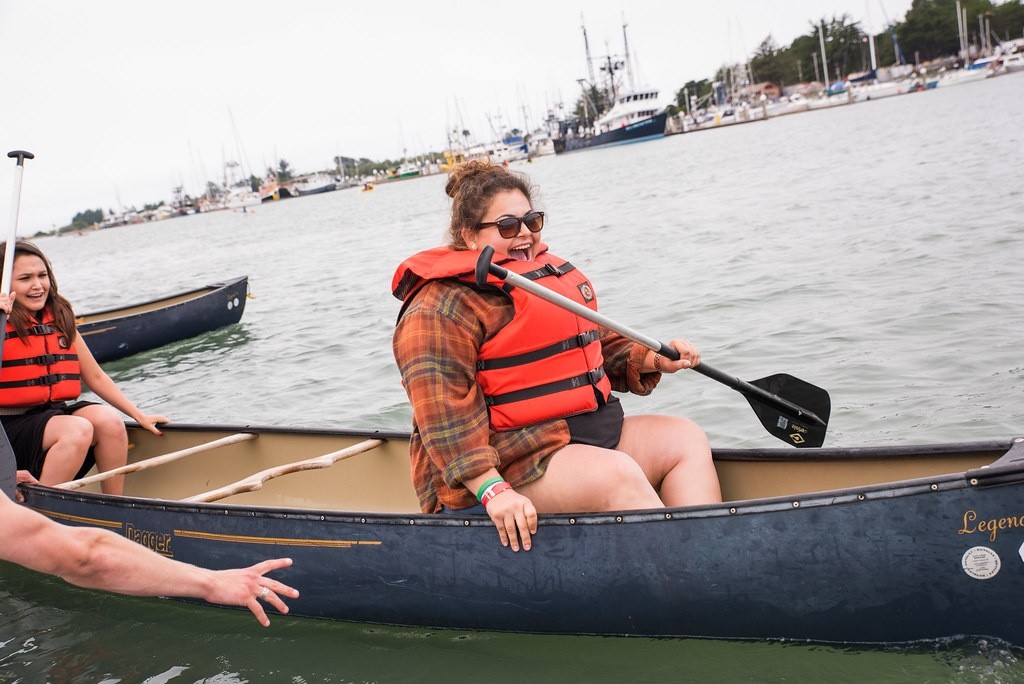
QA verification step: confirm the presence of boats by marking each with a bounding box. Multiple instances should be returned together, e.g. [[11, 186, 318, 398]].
[[15, 418, 1024, 653], [98, 15, 1024, 226], [75, 274, 250, 364]]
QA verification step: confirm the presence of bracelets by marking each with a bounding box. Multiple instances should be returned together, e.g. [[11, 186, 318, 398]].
[[654, 354, 663, 373], [477, 477, 512, 510]]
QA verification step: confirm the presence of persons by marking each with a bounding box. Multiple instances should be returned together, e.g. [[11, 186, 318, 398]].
[[0, 242, 169, 495], [393, 164, 723, 552], [0, 490, 300, 626]]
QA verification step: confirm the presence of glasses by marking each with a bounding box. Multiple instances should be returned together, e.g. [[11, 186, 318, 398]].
[[476, 212, 545, 239]]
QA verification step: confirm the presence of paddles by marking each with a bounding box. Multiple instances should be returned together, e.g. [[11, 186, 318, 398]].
[[474, 246, 831, 448], [0, 150, 34, 367]]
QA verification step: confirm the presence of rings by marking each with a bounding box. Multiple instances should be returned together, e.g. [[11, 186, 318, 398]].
[[260, 588, 269, 601]]
